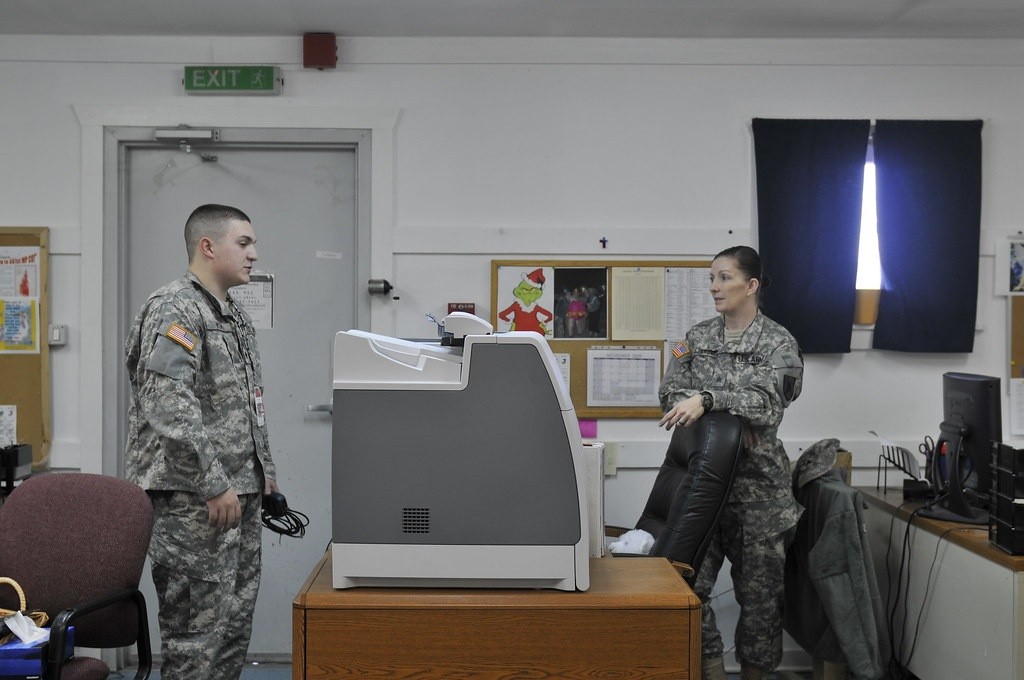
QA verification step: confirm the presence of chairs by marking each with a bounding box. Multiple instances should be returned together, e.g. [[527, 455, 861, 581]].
[[1, 473, 153, 680]]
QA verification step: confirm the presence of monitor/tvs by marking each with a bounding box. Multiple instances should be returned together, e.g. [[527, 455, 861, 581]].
[[917, 371, 1003, 524]]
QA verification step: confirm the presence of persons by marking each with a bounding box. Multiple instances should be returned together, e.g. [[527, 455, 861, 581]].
[[124, 203, 279, 680], [555, 284, 602, 339], [658, 245, 804, 680]]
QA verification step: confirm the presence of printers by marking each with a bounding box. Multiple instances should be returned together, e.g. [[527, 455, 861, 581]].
[[330, 311, 590, 593]]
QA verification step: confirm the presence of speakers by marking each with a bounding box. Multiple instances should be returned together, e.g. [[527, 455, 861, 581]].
[[303, 33, 336, 68]]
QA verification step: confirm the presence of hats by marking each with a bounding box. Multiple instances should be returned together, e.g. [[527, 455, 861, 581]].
[[793, 439, 841, 508]]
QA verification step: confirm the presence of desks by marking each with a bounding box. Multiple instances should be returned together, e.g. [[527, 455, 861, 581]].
[[851, 485, 1024, 679], [289, 554, 702, 679]]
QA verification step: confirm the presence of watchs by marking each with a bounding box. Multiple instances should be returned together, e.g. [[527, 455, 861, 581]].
[[700, 392, 714, 414]]
